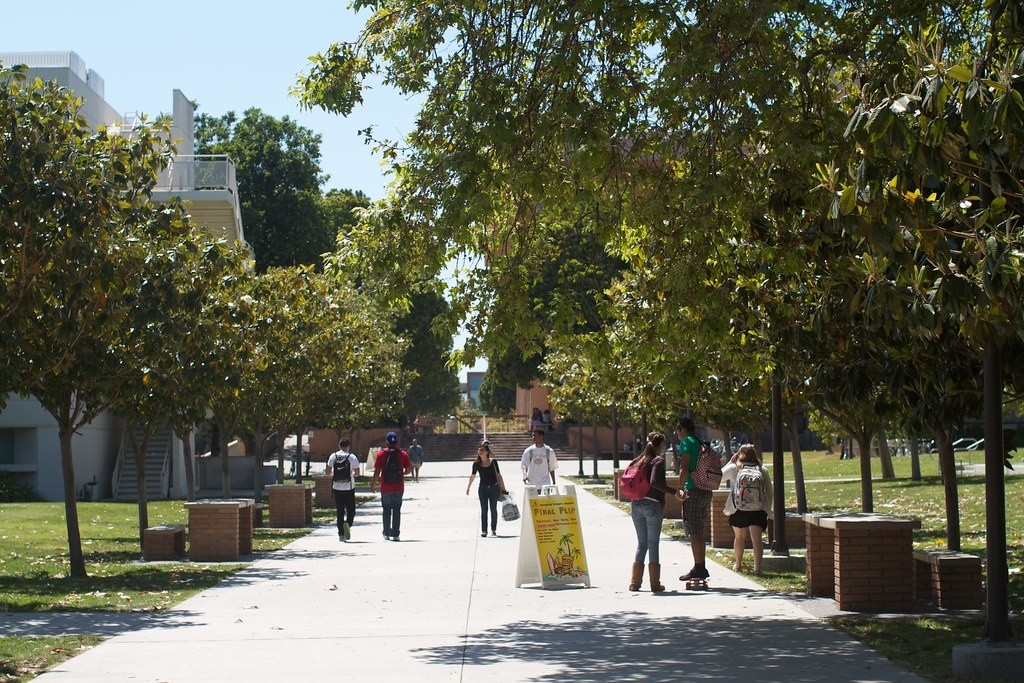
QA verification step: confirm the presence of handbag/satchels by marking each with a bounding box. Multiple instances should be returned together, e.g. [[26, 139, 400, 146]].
[[502, 495, 520, 521], [498, 489, 503, 501]]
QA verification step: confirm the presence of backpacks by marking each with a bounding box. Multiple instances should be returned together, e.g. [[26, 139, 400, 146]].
[[382, 448, 403, 483], [685, 435, 723, 491], [620, 452, 660, 500], [732, 461, 770, 511], [333, 452, 352, 483]]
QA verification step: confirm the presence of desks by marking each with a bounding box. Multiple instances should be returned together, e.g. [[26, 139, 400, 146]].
[[264, 484, 314, 528], [313, 473, 359, 509], [801, 512, 920, 611], [708, 489, 736, 548], [183, 498, 256, 561], [665, 476, 683, 520], [614, 468, 631, 502]]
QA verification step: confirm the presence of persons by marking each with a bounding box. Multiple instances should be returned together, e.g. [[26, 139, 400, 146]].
[[676, 418, 713, 581], [409, 439, 424, 483], [371, 432, 412, 541], [527, 408, 553, 432], [520, 429, 559, 495], [327, 437, 360, 542], [720, 444, 773, 575], [628, 432, 687, 592], [466, 444, 505, 537], [836, 436, 856, 459]]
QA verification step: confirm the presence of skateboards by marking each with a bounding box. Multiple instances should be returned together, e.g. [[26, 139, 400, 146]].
[[685, 578, 708, 590]]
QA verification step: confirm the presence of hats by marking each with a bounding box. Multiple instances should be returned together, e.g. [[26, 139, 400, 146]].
[[386, 431, 398, 445]]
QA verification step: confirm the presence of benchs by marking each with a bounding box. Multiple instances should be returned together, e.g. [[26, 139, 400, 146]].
[[768, 513, 805, 548], [253, 503, 263, 525], [913, 548, 983, 609], [143, 524, 185, 561], [532, 424, 551, 431]]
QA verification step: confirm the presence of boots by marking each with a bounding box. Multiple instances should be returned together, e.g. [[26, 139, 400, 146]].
[[649, 564, 665, 592], [629, 562, 645, 591]]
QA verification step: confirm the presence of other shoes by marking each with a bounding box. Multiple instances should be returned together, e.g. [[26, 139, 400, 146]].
[[733, 563, 741, 572], [754, 570, 760, 576], [383, 534, 390, 540], [679, 567, 709, 581], [342, 523, 350, 540], [481, 531, 487, 537], [393, 536, 400, 541], [339, 534, 345, 542], [492, 529, 496, 536]]
